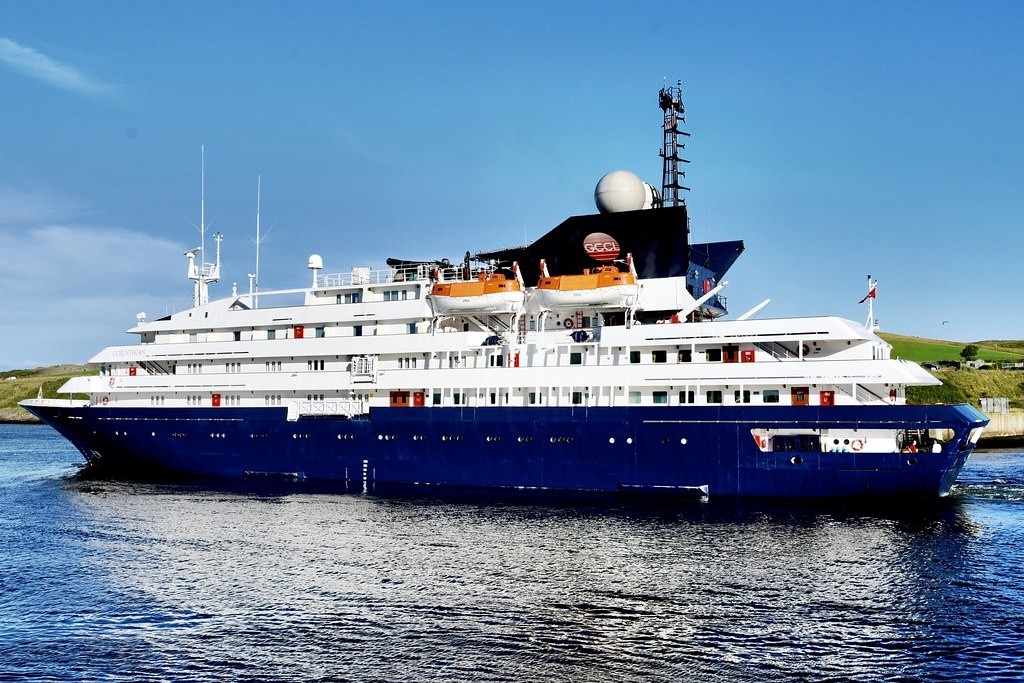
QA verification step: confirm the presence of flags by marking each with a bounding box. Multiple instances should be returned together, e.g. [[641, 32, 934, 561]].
[[858, 280, 878, 303]]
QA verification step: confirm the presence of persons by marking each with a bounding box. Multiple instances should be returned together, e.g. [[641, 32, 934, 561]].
[[107, 365, 112, 376], [908, 439, 918, 453], [932, 439, 942, 453], [101, 363, 107, 375]]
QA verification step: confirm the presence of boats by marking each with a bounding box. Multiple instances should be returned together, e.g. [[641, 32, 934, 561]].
[[532, 264, 644, 314], [424, 261, 529, 316], [17, 76, 991, 512]]
